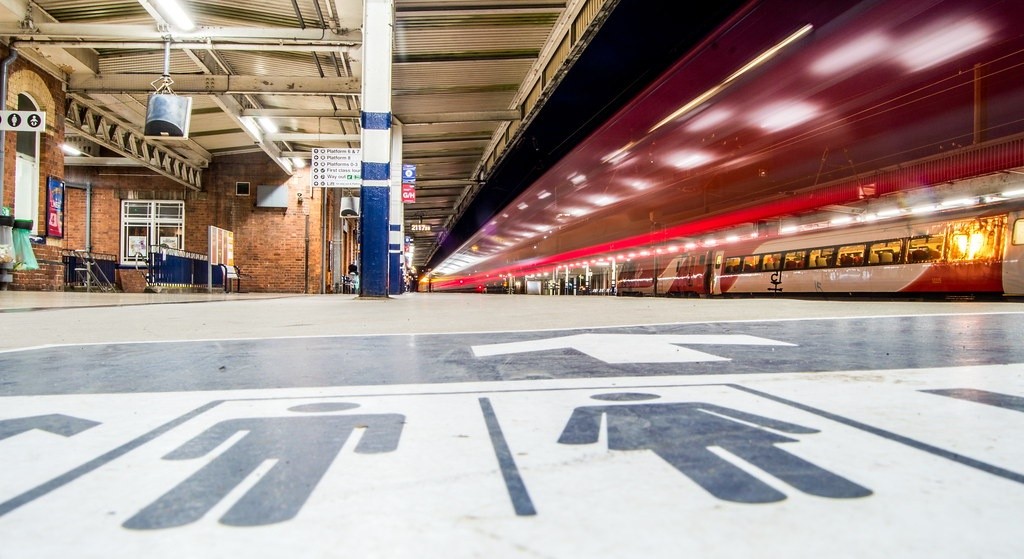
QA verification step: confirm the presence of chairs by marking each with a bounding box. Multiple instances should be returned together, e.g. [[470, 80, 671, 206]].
[[726, 249, 948, 272]]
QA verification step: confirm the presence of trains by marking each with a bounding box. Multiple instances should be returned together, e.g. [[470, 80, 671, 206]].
[[591, 195, 1023, 301]]
